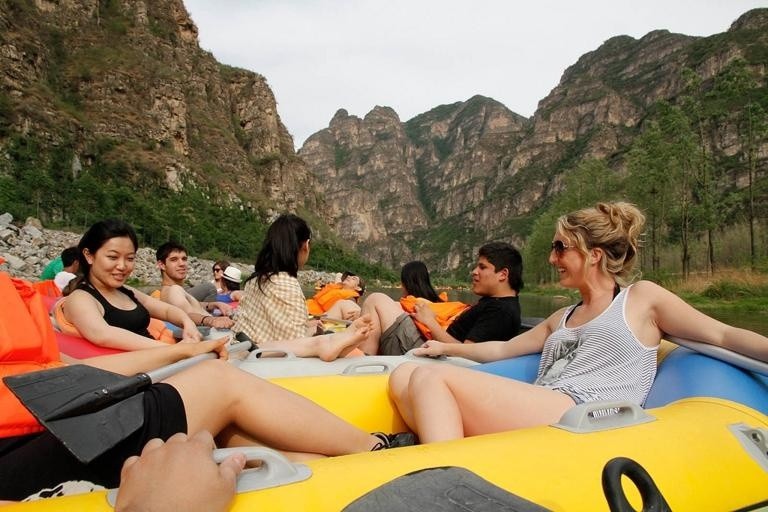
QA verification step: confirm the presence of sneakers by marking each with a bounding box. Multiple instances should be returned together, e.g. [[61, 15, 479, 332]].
[[371, 432, 415, 451]]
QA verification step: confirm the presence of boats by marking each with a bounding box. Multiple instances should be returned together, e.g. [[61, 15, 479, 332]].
[[0, 321, 767, 512]]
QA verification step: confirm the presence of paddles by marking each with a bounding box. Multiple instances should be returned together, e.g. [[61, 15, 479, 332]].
[[2, 333, 262, 464]]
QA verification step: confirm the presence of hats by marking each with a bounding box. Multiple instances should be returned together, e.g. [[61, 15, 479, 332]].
[[341, 270, 365, 296], [220, 266, 242, 284]]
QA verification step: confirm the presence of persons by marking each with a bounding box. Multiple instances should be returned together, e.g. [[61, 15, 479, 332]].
[[1, 256, 418, 511], [229, 215, 365, 360], [352, 242, 525, 356], [57, 218, 376, 361], [112, 429, 248, 510], [386, 199, 767, 445], [38, 243, 446, 327]]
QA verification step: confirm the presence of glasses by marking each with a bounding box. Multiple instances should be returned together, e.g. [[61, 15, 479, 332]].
[[551, 240, 593, 256], [214, 269, 220, 272]]
